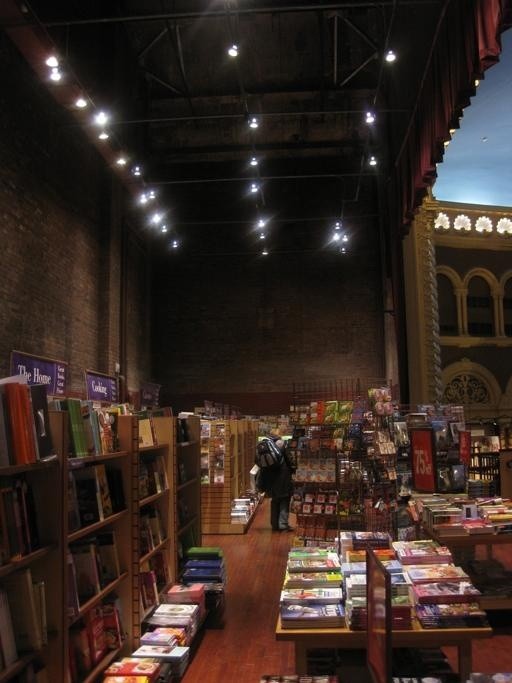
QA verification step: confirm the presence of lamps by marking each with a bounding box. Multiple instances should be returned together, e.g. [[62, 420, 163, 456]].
[[259, 232, 266, 240], [342, 234, 350, 243], [332, 233, 341, 242], [334, 222, 341, 231], [132, 165, 142, 177], [48, 67, 63, 82], [249, 154, 259, 166], [92, 111, 109, 127], [151, 214, 162, 224], [249, 117, 259, 128], [365, 111, 375, 124], [369, 156, 377, 166], [98, 128, 109, 141], [172, 240, 178, 248], [261, 250, 269, 256], [161, 226, 168, 233], [385, 50, 397, 64], [140, 194, 148, 205], [340, 247, 346, 254], [74, 91, 88, 109], [227, 43, 238, 58], [44, 54, 59, 67], [115, 154, 128, 165], [257, 219, 265, 228], [251, 183, 258, 193], [149, 190, 156, 200]]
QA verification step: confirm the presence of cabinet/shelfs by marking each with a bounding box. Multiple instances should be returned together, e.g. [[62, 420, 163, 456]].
[[174, 414, 202, 583], [399, 402, 471, 528], [0, 397, 63, 681], [62, 394, 136, 683], [135, 416, 175, 652], [290, 376, 398, 536], [203, 415, 266, 535]]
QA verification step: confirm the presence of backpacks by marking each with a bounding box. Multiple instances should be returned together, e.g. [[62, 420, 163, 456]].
[[256, 436, 284, 467]]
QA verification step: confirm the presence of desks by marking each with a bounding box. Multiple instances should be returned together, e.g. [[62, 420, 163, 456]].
[[417, 516, 512, 610], [274, 531, 493, 683]]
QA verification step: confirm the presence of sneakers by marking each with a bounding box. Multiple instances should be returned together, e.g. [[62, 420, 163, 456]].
[[280, 526, 294, 533]]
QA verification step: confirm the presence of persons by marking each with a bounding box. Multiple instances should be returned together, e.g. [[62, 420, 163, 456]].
[[255, 429, 299, 533]]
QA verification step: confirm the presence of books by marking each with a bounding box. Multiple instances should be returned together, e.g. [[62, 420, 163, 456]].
[[140, 548, 172, 608], [406, 494, 512, 536], [153, 405, 173, 417], [152, 627, 189, 647], [161, 584, 206, 622], [137, 410, 158, 448], [68, 464, 125, 532], [305, 650, 342, 677], [0, 374, 51, 466], [334, 531, 415, 631], [50, 395, 120, 457], [0, 477, 41, 566], [467, 559, 512, 600], [183, 560, 227, 568], [69, 595, 129, 683], [118, 656, 176, 683], [230, 490, 259, 525], [145, 617, 192, 645], [177, 582, 225, 610], [280, 547, 347, 629], [102, 676, 149, 683], [185, 547, 224, 560], [67, 526, 122, 621], [416, 648, 452, 674], [24, 663, 51, 683], [140, 633, 179, 647], [140, 456, 169, 500], [152, 604, 200, 637], [391, 539, 488, 629], [182, 568, 226, 585], [103, 662, 160, 681], [464, 479, 490, 497], [139, 504, 165, 554], [0, 568, 49, 670], [131, 645, 190, 678]]
